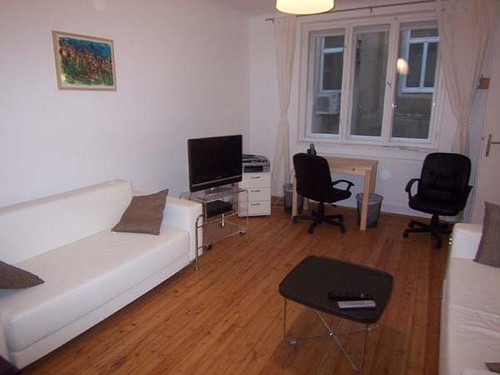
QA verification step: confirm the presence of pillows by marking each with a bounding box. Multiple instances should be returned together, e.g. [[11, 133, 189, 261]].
[[473, 201, 500, 267], [110, 188, 170, 235], [0, 260, 44, 290], [485, 361, 500, 373]]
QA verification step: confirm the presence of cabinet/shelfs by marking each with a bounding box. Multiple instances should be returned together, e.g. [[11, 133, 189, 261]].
[[235, 172, 273, 218], [182, 188, 248, 247]]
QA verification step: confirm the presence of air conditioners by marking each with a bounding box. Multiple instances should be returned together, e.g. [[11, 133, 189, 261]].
[[314, 91, 341, 115]]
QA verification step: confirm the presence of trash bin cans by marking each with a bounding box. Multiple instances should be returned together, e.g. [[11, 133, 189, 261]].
[[355, 193, 383, 228], [282, 183, 303, 212]]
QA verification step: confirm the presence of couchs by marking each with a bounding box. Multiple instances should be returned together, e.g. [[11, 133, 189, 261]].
[[439, 222, 500, 374], [0, 179, 206, 370]]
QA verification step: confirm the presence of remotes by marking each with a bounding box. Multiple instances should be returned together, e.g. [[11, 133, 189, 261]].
[[337, 300, 377, 310], [328, 292, 367, 299]]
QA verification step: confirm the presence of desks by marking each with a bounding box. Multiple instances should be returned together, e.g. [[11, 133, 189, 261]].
[[291, 153, 380, 232]]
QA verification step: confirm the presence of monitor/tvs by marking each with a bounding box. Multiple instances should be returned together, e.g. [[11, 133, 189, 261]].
[[187, 134, 243, 193]]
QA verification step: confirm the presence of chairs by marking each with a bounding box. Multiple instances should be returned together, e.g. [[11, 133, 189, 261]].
[[402, 151, 473, 248], [291, 152, 356, 234]]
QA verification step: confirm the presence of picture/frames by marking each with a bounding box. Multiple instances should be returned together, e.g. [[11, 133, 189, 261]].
[[51, 30, 117, 92]]
